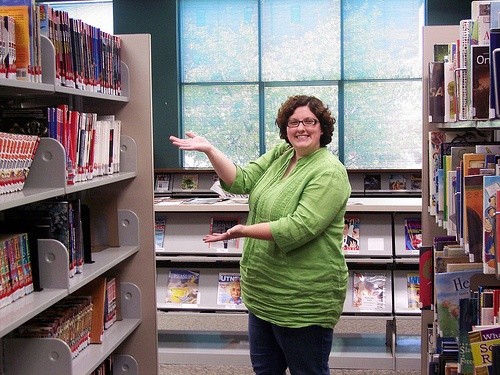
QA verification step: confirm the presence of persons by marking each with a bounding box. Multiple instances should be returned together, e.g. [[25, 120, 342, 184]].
[[169, 95, 352, 375]]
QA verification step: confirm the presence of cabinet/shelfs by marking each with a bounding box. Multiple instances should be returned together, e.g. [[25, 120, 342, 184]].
[[0, 34, 159, 375], [154, 167, 423, 369], [421, 25, 500, 375]]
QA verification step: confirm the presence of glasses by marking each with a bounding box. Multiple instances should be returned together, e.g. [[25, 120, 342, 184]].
[[287, 117, 319, 127]]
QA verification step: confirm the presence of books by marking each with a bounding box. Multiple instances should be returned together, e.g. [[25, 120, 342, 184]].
[[153, 170, 423, 310], [0, 0, 124, 375], [420, 0, 500, 375]]
